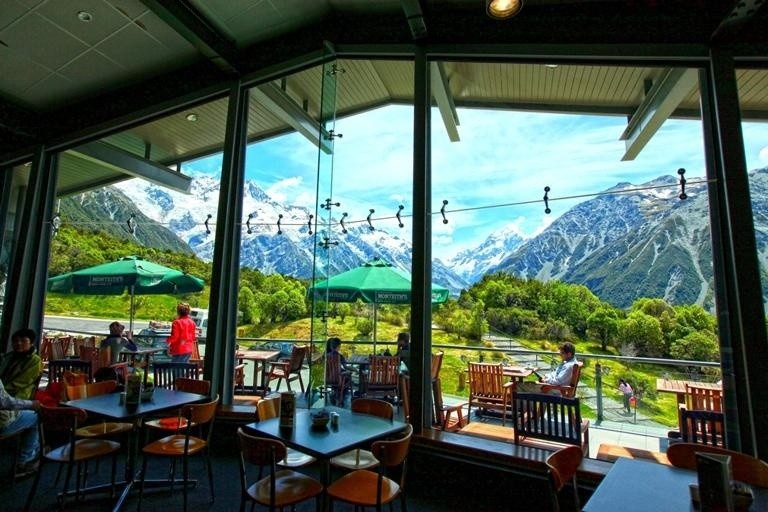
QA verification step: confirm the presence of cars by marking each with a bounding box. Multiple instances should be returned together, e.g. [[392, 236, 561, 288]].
[[133, 328, 175, 365], [247, 341, 307, 372]]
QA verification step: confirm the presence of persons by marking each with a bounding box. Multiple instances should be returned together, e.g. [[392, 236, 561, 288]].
[[397, 333, 412, 404], [514, 342, 578, 418], [166, 303, 196, 379], [100, 322, 137, 374], [0, 379, 41, 479], [321, 338, 333, 354], [325, 338, 357, 406], [0, 328, 43, 400], [618, 378, 634, 412]]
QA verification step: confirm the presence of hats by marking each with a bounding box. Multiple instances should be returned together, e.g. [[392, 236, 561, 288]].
[[109, 321, 124, 335]]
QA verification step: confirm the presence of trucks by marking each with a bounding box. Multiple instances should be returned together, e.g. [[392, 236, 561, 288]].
[[188, 307, 209, 343]]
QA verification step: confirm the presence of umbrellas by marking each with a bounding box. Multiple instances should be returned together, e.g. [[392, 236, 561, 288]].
[[306, 258, 449, 356], [47, 256, 204, 336]]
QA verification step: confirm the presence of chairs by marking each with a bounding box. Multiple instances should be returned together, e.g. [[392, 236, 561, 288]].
[[25, 398, 119, 512], [36, 331, 306, 443], [327, 424, 414, 512], [136, 394, 219, 512], [655, 375, 726, 462], [545, 444, 583, 512], [665, 443, 767, 489], [305, 342, 591, 459], [235, 427, 325, 512]]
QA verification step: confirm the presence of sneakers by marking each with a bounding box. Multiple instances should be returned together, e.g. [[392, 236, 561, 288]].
[[15, 459, 40, 478]]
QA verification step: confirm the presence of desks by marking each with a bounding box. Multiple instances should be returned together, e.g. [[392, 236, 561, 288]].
[[59, 388, 205, 511], [246, 405, 408, 512], [581, 456, 767, 512]]
